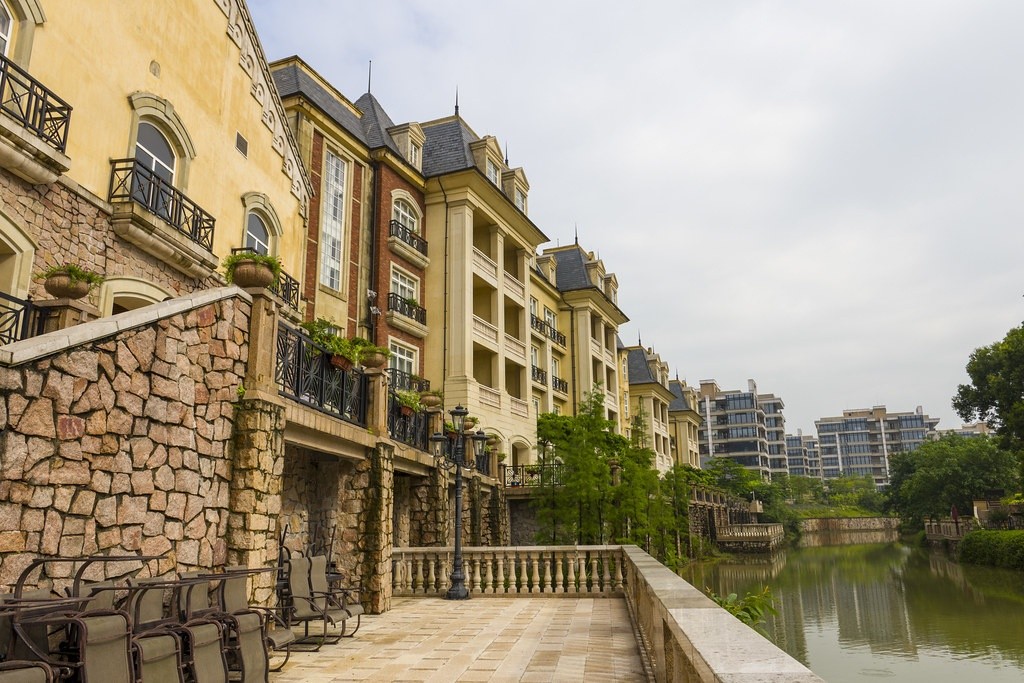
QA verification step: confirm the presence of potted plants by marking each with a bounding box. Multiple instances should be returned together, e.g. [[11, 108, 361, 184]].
[[419, 389, 445, 406], [410, 229, 420, 240], [397, 390, 422, 417], [444, 426, 457, 439], [464, 416, 480, 430], [324, 337, 359, 373], [406, 299, 419, 308], [486, 434, 500, 445], [355, 344, 393, 367], [221, 249, 282, 287], [33, 262, 105, 300], [525, 466, 539, 474], [497, 452, 507, 463]]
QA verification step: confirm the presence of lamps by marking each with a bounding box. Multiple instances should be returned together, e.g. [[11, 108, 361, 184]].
[[366, 287, 381, 315]]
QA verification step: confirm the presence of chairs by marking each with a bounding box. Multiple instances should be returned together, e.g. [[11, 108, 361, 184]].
[[0, 523, 366, 683]]
